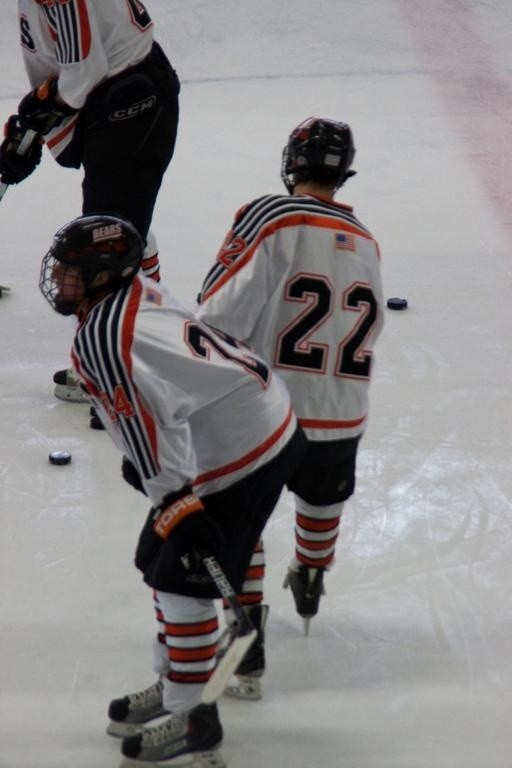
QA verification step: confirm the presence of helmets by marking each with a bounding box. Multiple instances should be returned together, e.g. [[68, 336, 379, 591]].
[[281, 117, 353, 194], [39, 216, 143, 316]]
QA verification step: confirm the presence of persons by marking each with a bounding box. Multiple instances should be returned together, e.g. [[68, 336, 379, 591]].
[[197, 115, 386, 676], [0, 1, 182, 389], [37, 214, 306, 760]]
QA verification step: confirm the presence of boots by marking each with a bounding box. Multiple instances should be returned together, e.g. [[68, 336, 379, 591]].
[[288, 558, 324, 617], [217, 604, 265, 677]]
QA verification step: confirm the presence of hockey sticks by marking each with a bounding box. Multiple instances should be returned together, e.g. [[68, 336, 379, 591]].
[[1, 126, 37, 291], [201, 556, 258, 704]]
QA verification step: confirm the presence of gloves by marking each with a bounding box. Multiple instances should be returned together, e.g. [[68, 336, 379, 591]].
[[18, 77, 79, 135], [0, 115, 43, 185], [121, 456, 148, 498], [150, 491, 226, 575]]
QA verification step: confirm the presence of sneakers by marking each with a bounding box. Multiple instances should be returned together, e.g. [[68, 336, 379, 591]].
[[54, 369, 80, 386], [108, 681, 170, 723], [122, 702, 221, 761]]
